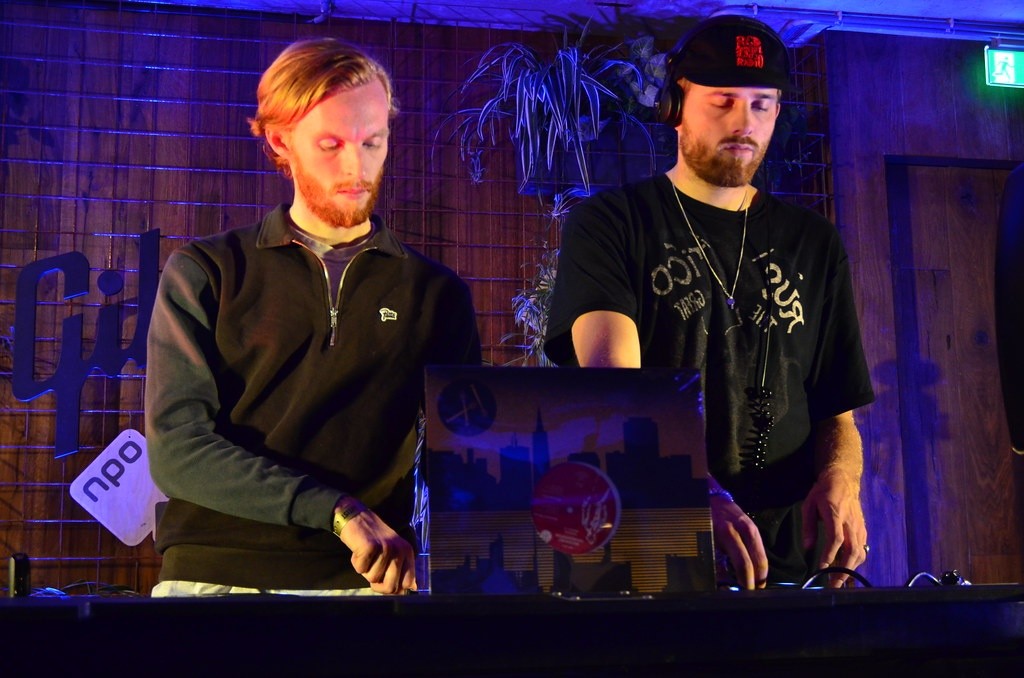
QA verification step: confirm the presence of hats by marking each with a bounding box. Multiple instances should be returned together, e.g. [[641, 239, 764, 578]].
[[675, 20, 787, 90]]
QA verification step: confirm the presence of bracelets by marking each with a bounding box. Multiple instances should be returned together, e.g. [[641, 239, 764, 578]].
[[334, 502, 369, 537], [708, 488, 729, 496]]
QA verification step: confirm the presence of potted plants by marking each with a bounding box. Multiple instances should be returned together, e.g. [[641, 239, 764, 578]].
[[429, 4, 678, 197]]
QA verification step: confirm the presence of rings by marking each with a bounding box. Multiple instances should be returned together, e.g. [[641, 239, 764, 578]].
[[864, 545, 870, 553]]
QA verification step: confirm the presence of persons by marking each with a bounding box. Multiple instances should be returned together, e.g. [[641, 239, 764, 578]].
[[143, 36, 481, 599], [543, 15, 875, 591]]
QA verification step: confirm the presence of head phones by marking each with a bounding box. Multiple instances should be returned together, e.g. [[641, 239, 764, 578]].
[[654, 16, 793, 128]]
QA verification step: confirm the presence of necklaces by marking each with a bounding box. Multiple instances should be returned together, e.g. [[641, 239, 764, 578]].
[[671, 166, 749, 309]]
